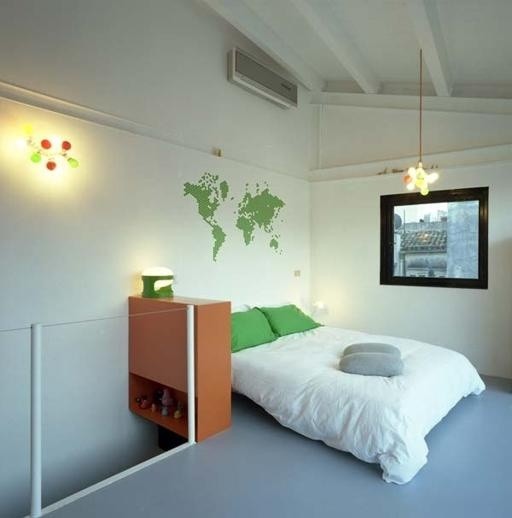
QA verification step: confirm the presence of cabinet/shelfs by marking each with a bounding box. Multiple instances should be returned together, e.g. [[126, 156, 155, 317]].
[[128, 297, 233, 443]]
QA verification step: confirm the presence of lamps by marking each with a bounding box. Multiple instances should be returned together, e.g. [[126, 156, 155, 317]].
[[401, 49, 440, 197], [23, 123, 82, 178]]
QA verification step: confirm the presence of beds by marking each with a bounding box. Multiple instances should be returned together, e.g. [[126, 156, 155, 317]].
[[225, 298, 486, 485]]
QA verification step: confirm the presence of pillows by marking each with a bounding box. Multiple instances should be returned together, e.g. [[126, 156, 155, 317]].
[[341, 341, 402, 376], [230, 307, 276, 354], [259, 304, 320, 337]]
